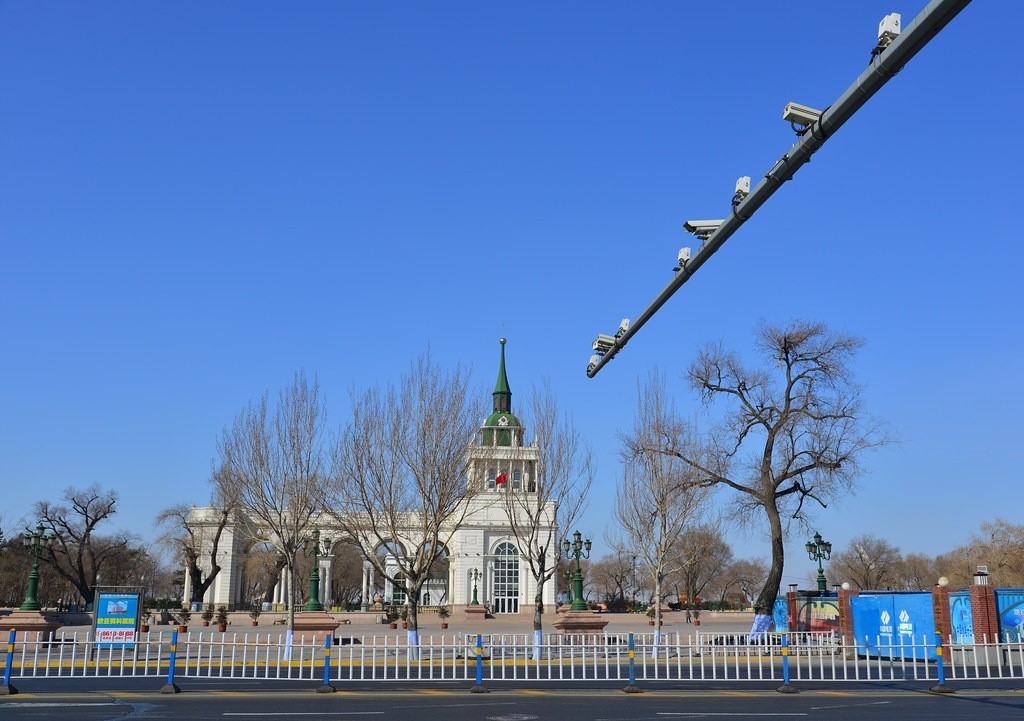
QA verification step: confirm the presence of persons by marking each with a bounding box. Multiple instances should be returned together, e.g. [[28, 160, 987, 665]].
[[685, 608, 692, 624], [484, 600, 491, 614]]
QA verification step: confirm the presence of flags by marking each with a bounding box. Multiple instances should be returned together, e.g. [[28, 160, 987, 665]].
[[496, 472, 508, 486]]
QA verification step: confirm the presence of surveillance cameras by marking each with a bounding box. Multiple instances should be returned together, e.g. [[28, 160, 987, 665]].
[[592, 334, 621, 352], [683, 220, 726, 240], [783, 102, 822, 127]]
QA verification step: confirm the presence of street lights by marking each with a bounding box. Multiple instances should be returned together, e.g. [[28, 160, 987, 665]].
[[19, 522, 57, 610], [301, 527, 331, 611], [563, 568, 574, 604], [805, 530, 832, 593], [563, 529, 593, 610], [468, 567, 483, 604]]
